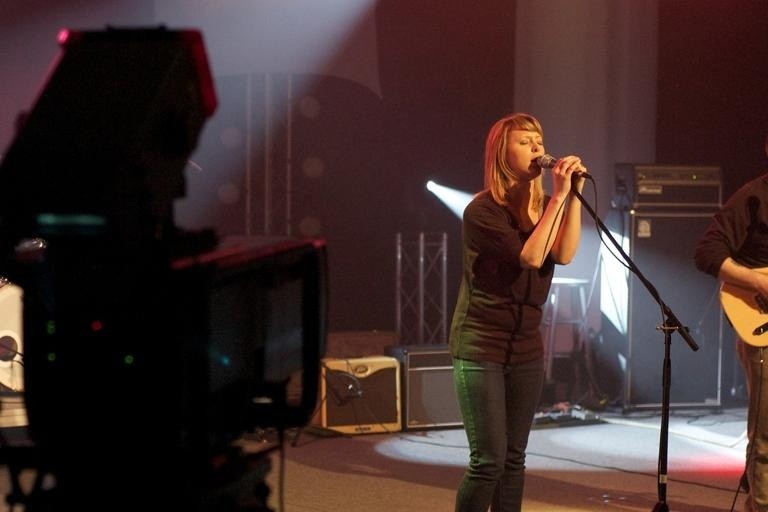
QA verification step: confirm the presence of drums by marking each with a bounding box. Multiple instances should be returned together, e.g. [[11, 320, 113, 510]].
[[0, 277, 25, 394]]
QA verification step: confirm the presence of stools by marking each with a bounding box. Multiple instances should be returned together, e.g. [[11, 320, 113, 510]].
[[541, 277, 602, 394]]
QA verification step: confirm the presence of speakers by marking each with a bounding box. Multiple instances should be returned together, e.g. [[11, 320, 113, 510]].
[[383, 343, 464, 431], [600, 207, 725, 411], [319, 354, 402, 435]]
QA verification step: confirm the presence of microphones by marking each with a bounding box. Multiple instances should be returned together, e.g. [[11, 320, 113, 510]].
[[535, 153, 591, 179]]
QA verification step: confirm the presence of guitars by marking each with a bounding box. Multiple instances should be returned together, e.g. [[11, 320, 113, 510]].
[[719, 267, 768, 348]]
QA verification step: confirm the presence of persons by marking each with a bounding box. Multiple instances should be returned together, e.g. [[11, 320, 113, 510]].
[[693, 173, 767, 512], [445, 112, 590, 512]]
